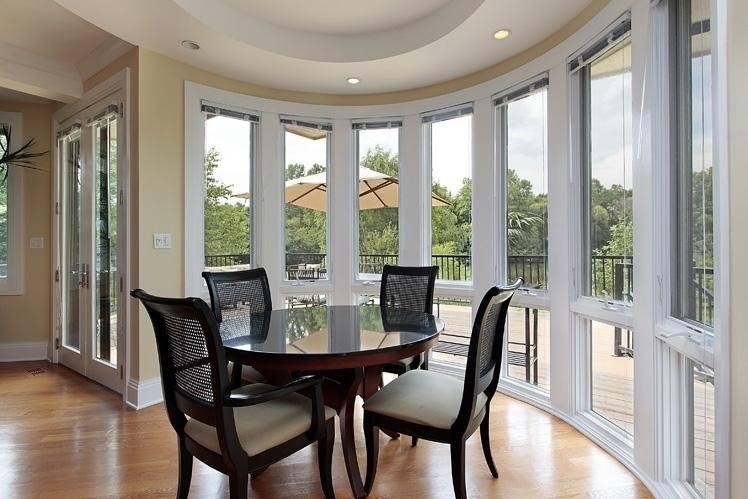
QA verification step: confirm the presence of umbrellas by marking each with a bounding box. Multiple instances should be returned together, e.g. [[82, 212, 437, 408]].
[[227, 163, 454, 215]]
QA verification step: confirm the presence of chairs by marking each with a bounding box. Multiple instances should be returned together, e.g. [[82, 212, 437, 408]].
[[201, 266, 283, 385], [380, 266, 441, 447], [361, 277, 525, 498], [132, 288, 336, 497]]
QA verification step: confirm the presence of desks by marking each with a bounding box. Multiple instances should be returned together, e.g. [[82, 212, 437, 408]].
[[218, 305, 444, 498]]
